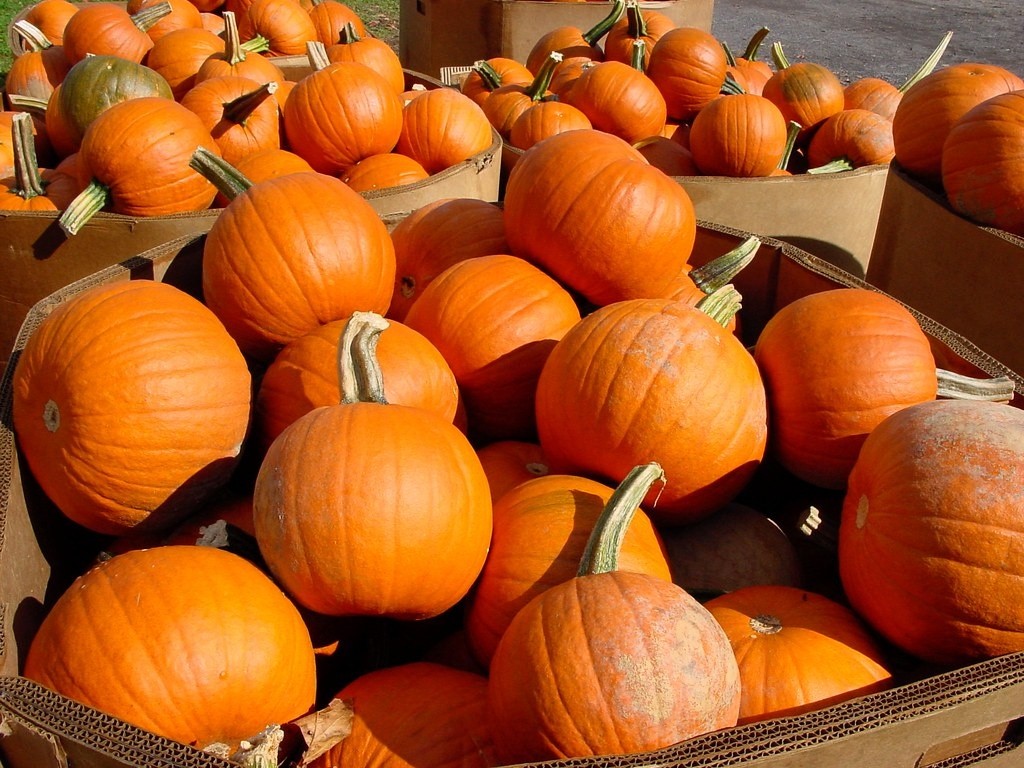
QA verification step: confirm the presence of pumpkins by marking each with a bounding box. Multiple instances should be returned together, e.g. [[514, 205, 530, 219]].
[[1, 0, 1024, 768]]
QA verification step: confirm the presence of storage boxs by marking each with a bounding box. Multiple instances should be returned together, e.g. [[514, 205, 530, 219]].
[[0, 0, 1024, 768]]
